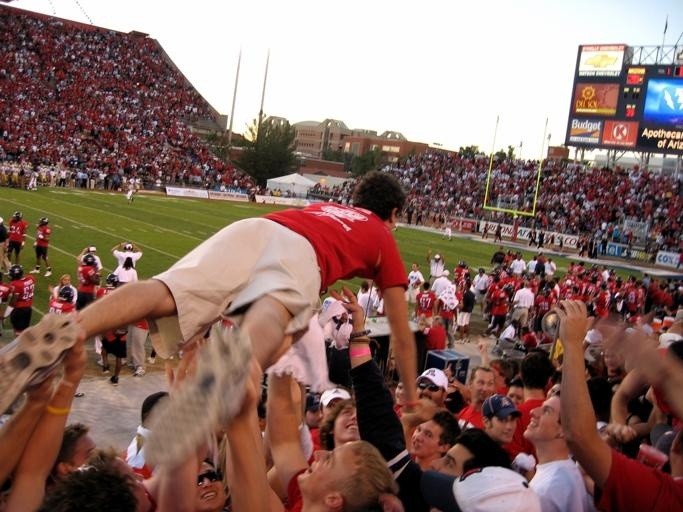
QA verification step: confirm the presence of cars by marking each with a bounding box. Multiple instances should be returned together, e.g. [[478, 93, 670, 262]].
[[637, 443, 668, 471]]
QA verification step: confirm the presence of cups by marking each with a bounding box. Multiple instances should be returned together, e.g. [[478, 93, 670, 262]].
[[417, 382, 439, 392], [197, 471, 217, 486]]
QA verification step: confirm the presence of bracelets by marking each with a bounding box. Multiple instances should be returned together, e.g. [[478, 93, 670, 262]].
[[349, 338, 371, 343], [350, 331, 367, 338], [49, 406, 71, 414], [349, 347, 371, 358]]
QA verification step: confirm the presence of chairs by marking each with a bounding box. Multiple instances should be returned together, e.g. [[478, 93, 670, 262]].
[[137, 330, 251, 465], [29, 269, 39, 273], [43, 271, 51, 277], [0, 313, 79, 416], [97, 356, 155, 376]]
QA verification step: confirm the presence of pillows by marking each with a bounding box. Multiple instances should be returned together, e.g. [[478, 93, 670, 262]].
[[458, 260, 465, 265], [7, 264, 22, 278], [12, 211, 22, 221], [59, 285, 73, 302], [39, 217, 48, 225], [81, 255, 95, 264], [106, 274, 118, 284]]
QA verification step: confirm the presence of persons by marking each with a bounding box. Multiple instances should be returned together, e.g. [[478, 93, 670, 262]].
[[305, 146, 682, 240], [0, 216, 12, 273], [28, 217, 52, 276], [0, 1, 293, 205], [7, 212, 27, 266], [0, 240, 683, 512], [0, 171, 436, 476]]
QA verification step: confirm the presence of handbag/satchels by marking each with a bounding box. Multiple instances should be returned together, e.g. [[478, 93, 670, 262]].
[[401, 400, 424, 408]]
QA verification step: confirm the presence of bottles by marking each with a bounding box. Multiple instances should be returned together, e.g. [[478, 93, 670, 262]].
[[434, 252, 440, 258], [319, 388, 351, 407], [124, 244, 132, 251], [416, 367, 448, 391], [88, 246, 96, 252], [419, 466, 542, 511], [482, 394, 521, 418], [649, 422, 677, 452]]
